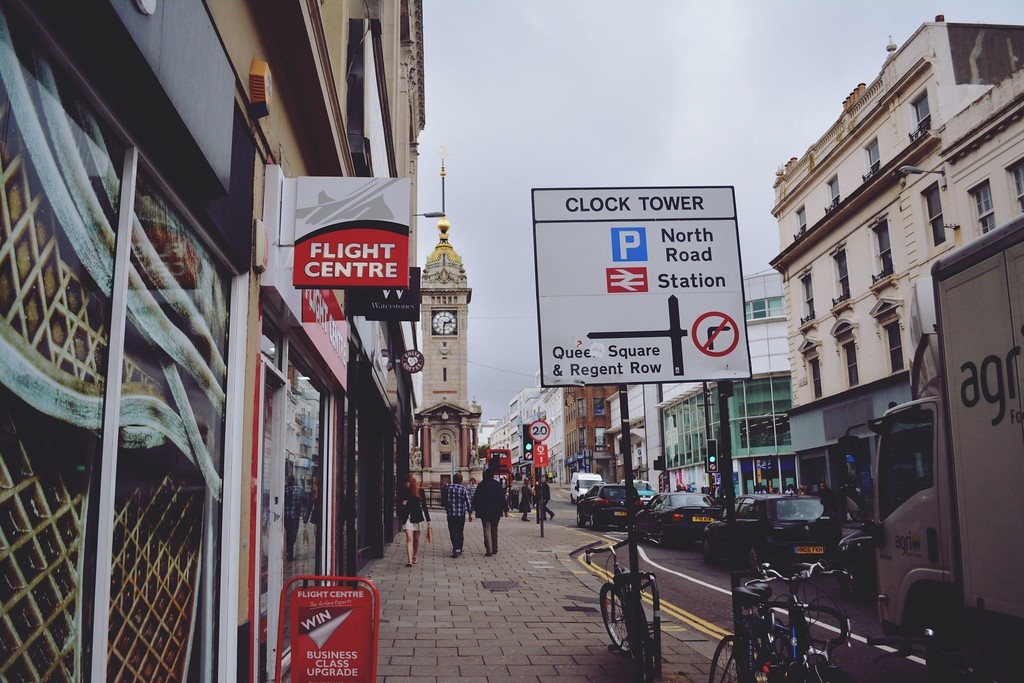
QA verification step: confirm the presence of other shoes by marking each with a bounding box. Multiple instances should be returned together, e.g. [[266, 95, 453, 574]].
[[453, 549, 460, 557], [412, 556, 417, 564], [522, 518, 529, 521], [485, 553, 492, 556], [540, 517, 547, 520], [286, 545, 294, 560], [492, 549, 497, 554], [406, 561, 413, 566], [550, 513, 555, 520]]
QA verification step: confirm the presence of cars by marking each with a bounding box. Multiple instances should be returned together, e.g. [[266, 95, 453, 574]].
[[636, 491, 720, 546], [707, 490, 842, 564], [575, 482, 641, 532]]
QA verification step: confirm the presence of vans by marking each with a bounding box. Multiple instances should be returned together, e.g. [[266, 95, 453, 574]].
[[620, 479, 656, 506], [570, 471, 603, 504]]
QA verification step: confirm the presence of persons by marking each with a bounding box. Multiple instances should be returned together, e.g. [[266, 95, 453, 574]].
[[473, 469, 510, 556], [753, 477, 856, 523], [411, 446, 423, 468], [398, 473, 432, 567], [713, 484, 721, 498], [470, 444, 480, 467], [466, 478, 476, 511], [529, 475, 536, 508], [498, 476, 511, 498], [674, 482, 696, 493], [442, 483, 450, 507], [534, 480, 541, 503], [520, 476, 532, 521], [443, 472, 472, 559], [514, 470, 556, 483], [538, 478, 555, 520]]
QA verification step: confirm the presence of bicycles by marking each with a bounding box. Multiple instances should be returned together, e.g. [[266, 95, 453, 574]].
[[582, 533, 659, 668], [710, 559, 1003, 683]]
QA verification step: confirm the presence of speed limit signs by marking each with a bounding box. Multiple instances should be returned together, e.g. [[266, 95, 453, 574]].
[[528, 420, 551, 442]]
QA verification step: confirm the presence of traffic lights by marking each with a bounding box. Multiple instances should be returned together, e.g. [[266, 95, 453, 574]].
[[653, 456, 663, 469], [705, 438, 717, 473], [522, 423, 534, 460]]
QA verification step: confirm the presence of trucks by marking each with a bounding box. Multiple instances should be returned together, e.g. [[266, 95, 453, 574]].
[[847, 216, 1024, 648]]
